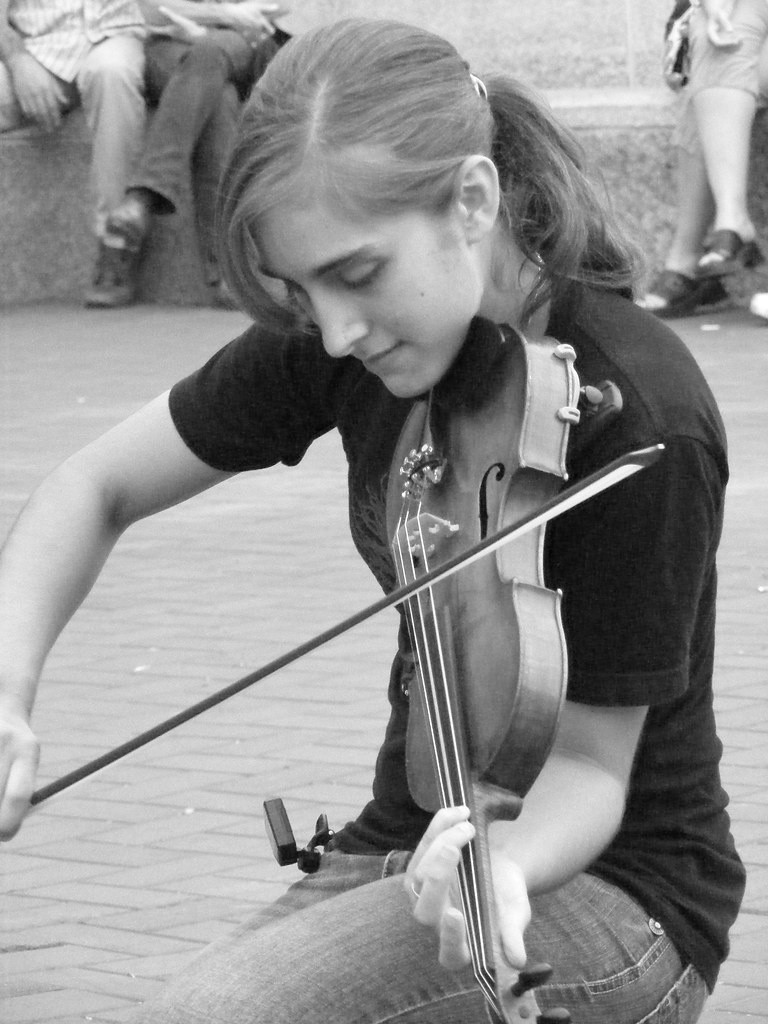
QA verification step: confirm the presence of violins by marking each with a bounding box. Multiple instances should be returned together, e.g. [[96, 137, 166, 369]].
[[385, 312, 622, 1024]]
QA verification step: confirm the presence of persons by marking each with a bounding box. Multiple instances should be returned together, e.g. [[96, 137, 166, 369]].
[[0, 1, 768, 320], [0, 22, 746, 1024]]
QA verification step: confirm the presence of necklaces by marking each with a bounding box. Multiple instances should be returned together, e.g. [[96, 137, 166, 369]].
[[519, 250, 542, 337]]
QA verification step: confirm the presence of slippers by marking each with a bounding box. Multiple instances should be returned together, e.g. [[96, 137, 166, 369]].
[[694, 226, 765, 278], [634, 269, 729, 320]]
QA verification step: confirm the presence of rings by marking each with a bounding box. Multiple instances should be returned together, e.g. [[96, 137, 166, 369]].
[[410, 883, 420, 899]]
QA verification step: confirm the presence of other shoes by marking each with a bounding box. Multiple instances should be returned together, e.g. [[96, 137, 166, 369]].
[[83, 256, 136, 306], [104, 190, 153, 255]]
